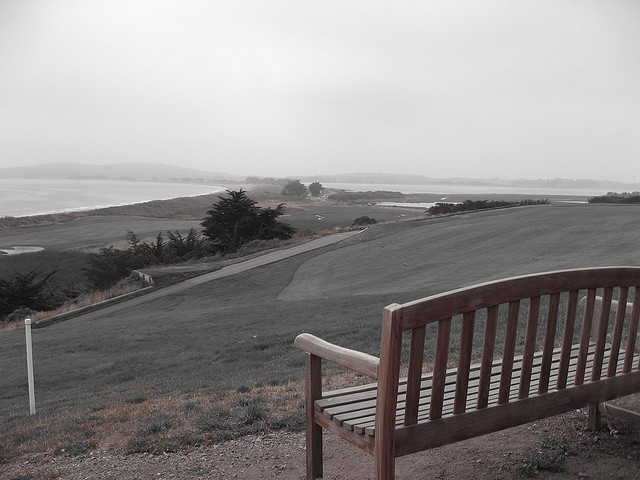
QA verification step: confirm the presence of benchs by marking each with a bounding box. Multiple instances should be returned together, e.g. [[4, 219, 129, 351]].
[[294, 265, 640, 480]]
